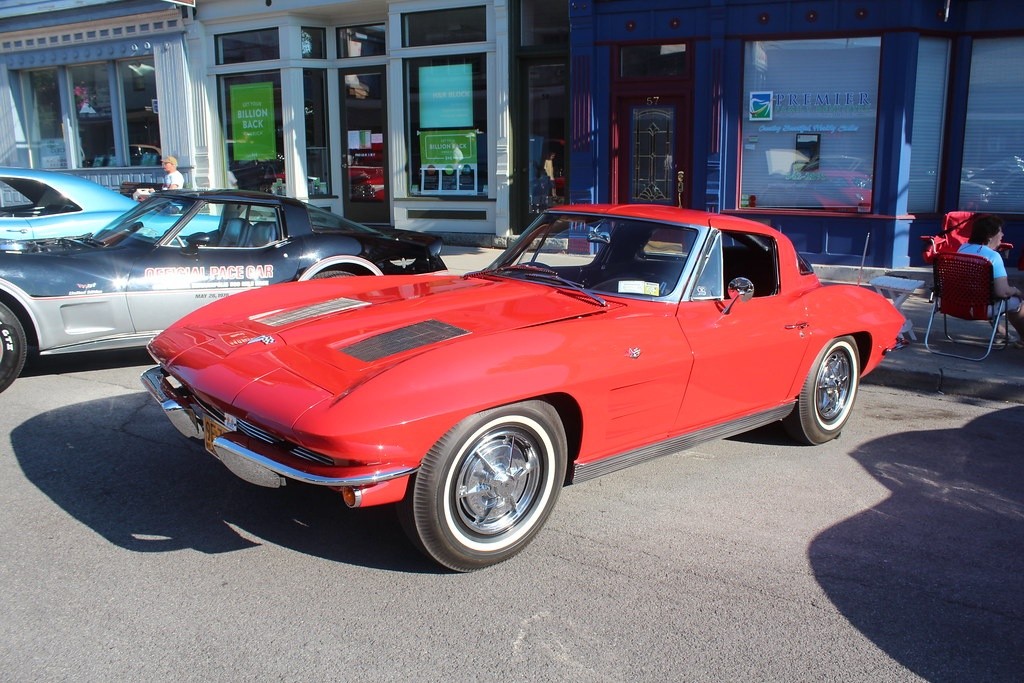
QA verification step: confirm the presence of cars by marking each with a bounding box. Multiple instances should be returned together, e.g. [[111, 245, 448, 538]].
[[752, 158, 1024, 211]]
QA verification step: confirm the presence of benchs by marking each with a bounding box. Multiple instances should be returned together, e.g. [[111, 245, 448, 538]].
[[120, 183, 164, 200]]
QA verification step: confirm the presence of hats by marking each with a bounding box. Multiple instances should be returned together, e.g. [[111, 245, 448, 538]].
[[160, 156, 177, 166]]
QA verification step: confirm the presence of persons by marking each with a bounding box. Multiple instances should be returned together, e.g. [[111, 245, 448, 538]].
[[955, 213, 1024, 343], [161, 156, 185, 189], [543, 150, 560, 201]]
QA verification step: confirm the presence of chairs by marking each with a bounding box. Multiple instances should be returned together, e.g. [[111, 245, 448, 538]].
[[216, 218, 251, 247], [920, 210, 1013, 303], [721, 245, 777, 298], [39, 203, 67, 215], [244, 221, 278, 248], [924, 253, 1011, 362]]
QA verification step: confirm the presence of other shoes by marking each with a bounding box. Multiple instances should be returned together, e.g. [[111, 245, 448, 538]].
[[994, 335, 1018, 345]]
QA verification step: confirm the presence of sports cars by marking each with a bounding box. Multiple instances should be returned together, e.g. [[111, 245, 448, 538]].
[[141, 203, 905, 573], [0, 199, 453, 388], [0, 166, 267, 250]]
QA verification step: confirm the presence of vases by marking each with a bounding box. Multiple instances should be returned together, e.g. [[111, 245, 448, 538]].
[[79, 102, 97, 114]]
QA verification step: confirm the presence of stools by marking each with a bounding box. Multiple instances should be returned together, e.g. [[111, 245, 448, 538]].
[[869, 277, 925, 342]]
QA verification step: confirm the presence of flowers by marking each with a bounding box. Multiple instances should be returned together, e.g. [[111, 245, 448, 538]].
[[73, 84, 98, 111]]
